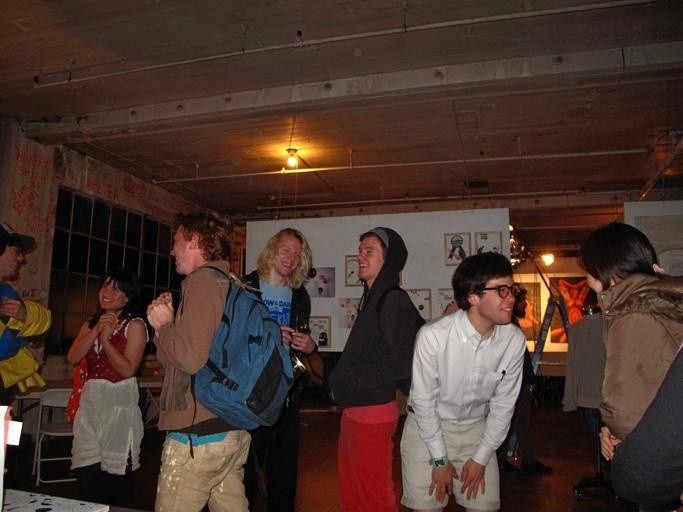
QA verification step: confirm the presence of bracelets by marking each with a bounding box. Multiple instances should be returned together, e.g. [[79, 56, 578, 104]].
[[306, 343, 318, 356]]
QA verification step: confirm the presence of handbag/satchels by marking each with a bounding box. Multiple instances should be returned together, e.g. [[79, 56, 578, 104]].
[[64, 358, 87, 423]]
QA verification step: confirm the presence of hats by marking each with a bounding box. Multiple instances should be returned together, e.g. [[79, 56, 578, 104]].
[[365, 228, 389, 249], [0, 223, 35, 249]]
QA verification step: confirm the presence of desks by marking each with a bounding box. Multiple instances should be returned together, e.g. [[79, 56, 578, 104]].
[[136, 374, 165, 429], [14, 378, 76, 435]]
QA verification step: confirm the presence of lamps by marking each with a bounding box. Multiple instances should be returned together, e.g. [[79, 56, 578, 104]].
[[286, 148, 298, 167], [529, 249, 555, 376]]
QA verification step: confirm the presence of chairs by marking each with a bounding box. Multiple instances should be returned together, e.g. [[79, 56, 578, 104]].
[[32, 388, 75, 486]]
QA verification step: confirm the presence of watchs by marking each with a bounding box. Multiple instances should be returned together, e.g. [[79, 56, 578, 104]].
[[428, 456, 448, 469]]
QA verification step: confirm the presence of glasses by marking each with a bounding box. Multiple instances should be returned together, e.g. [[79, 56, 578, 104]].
[[484, 282, 521, 298]]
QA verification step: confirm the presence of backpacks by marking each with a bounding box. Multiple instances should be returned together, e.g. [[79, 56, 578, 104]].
[[181, 266, 295, 433]]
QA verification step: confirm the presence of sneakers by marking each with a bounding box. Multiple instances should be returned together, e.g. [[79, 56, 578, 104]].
[[521, 459, 553, 474]]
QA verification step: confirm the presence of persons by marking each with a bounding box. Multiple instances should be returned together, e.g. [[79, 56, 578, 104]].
[[1, 218, 54, 421], [510, 287, 553, 476], [145, 207, 254, 512], [577, 221, 683, 511], [326, 226, 421, 512], [63, 266, 156, 508], [399, 253, 527, 512], [240, 227, 323, 512]]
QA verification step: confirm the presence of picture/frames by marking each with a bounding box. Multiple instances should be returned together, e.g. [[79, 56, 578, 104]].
[[309, 316, 331, 348], [444, 232, 471, 266], [473, 231, 503, 254], [404, 288, 432, 320], [345, 254, 364, 287], [435, 287, 460, 320]]
